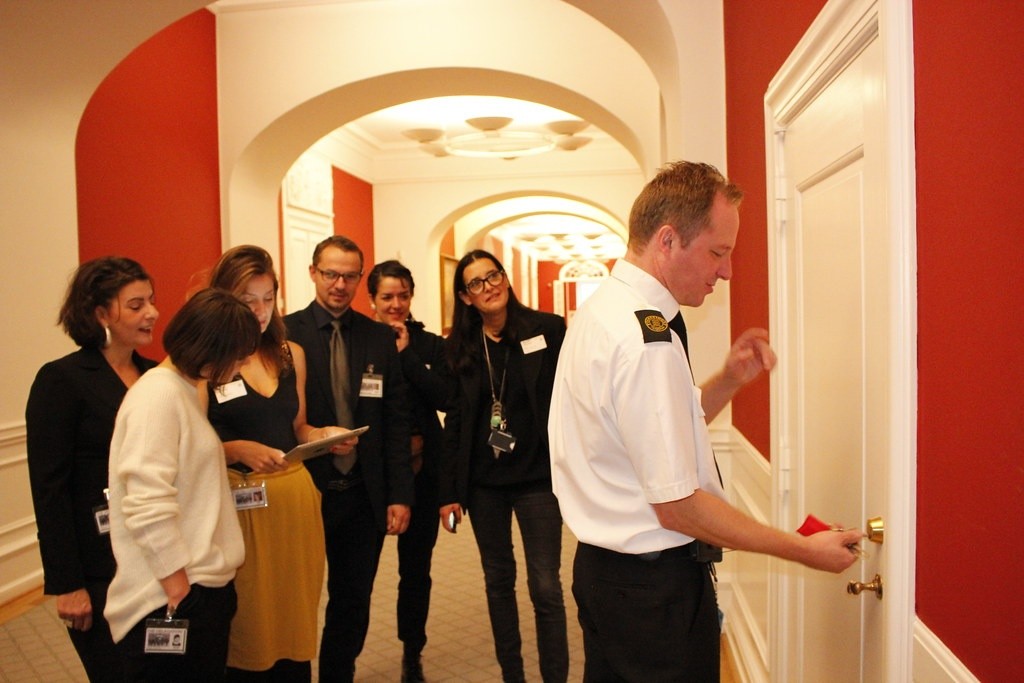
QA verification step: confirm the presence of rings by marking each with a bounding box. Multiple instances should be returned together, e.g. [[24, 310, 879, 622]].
[[62, 618, 74, 628]]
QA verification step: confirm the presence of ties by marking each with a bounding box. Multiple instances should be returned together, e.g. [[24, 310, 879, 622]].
[[668, 311, 724, 491], [329, 320, 363, 476]]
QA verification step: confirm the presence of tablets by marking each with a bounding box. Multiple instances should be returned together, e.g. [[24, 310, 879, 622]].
[[274, 425, 369, 469]]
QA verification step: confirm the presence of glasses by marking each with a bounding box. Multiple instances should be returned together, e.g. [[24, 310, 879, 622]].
[[464, 269, 504, 295], [314, 267, 361, 284]]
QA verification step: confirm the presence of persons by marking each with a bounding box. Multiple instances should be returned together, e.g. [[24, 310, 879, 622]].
[[548, 162, 862, 683], [254, 492, 261, 501], [25, 257, 160, 683], [102, 244, 452, 683], [440, 251, 568, 682], [173, 635, 181, 647]]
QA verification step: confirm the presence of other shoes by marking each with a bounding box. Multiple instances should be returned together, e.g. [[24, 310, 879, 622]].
[[401, 663, 424, 683]]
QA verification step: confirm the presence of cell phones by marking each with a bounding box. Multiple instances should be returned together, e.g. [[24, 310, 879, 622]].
[[449, 511, 457, 532]]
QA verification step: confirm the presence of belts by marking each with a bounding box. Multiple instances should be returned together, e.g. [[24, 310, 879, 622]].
[[314, 475, 363, 492], [640, 544, 697, 561]]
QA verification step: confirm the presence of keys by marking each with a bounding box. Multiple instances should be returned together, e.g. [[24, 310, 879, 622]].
[[835, 523, 873, 550]]
